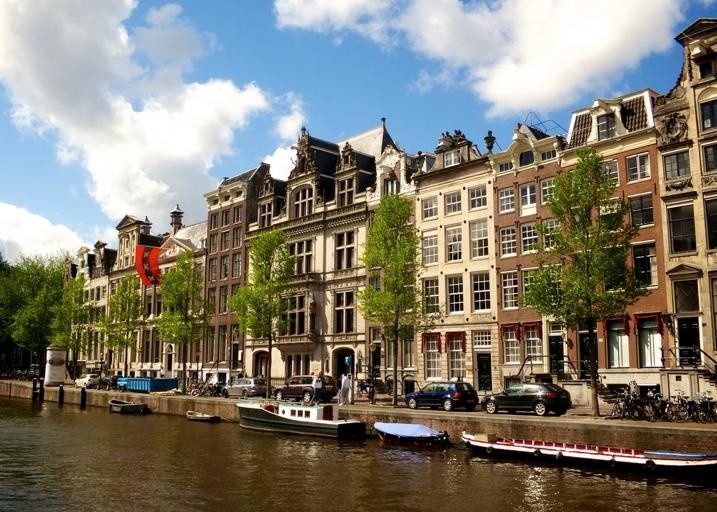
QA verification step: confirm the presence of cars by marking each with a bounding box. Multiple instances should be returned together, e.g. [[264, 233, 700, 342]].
[[74, 374, 123, 391], [221, 375, 337, 403], [404, 382, 572, 416]]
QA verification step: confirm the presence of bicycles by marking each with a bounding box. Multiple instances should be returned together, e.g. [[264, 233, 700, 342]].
[[610, 388, 717, 423]]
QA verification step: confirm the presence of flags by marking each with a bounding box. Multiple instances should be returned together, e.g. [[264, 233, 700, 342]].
[[135, 245, 162, 287]]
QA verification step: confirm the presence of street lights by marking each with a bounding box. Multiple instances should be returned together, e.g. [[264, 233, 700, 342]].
[[368, 342, 376, 404]]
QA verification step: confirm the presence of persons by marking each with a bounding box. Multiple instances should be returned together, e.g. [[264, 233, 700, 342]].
[[308, 371, 325, 406], [190, 379, 220, 397], [336, 371, 349, 405]]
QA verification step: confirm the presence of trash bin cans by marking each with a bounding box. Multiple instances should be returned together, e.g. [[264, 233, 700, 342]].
[[403, 379, 417, 395]]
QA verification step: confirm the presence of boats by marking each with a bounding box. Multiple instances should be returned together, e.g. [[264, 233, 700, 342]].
[[185, 410, 221, 421], [462, 429, 716, 483], [373, 421, 451, 447], [235, 404, 366, 440], [107, 398, 148, 414]]
